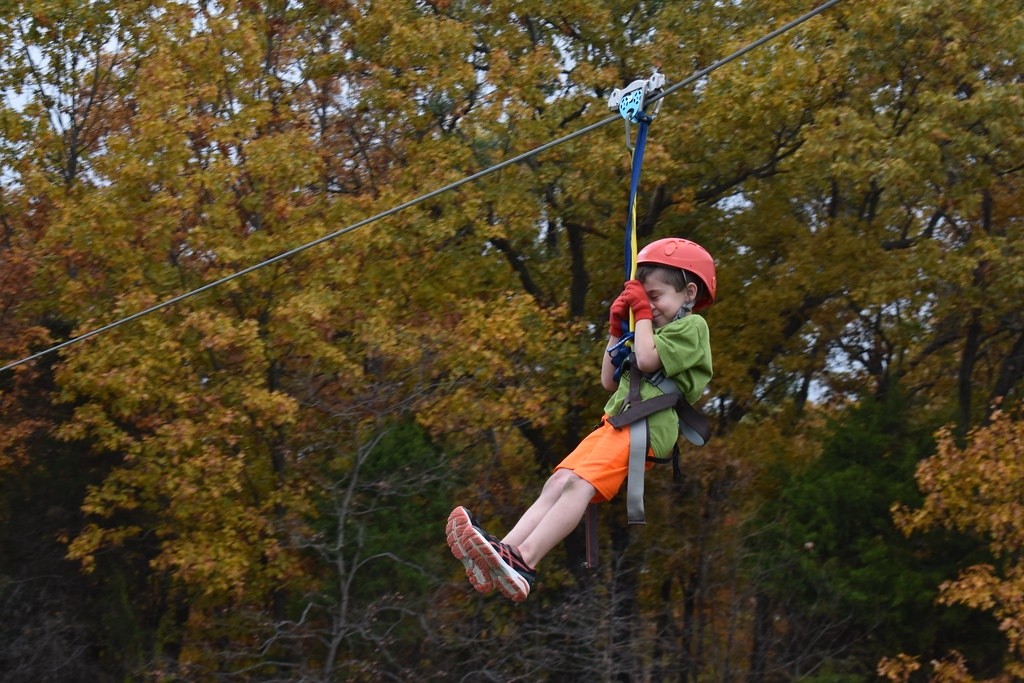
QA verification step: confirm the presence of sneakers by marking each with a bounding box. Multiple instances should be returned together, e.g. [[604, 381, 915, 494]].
[[462, 526, 536, 602], [446, 506, 495, 594]]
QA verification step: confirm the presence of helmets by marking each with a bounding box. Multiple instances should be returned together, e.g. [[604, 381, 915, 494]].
[[637, 237, 716, 309]]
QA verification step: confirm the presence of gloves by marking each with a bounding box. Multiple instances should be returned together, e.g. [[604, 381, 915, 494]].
[[624, 280, 653, 322], [609, 290, 626, 337]]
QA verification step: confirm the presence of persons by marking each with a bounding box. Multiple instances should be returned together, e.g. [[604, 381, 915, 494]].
[[445, 237, 718, 603]]
